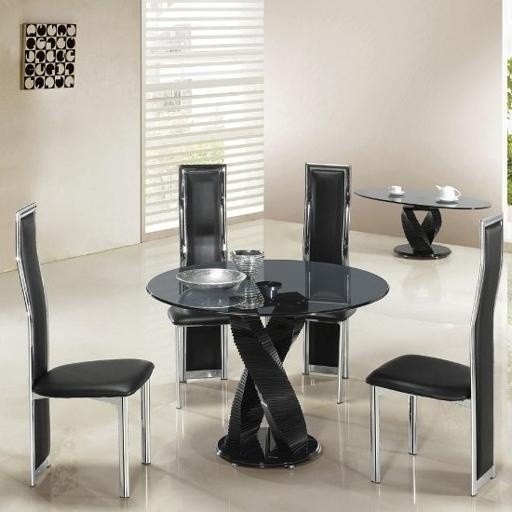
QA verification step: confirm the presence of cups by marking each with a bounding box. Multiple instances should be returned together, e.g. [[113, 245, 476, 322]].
[[391, 186, 401, 193]]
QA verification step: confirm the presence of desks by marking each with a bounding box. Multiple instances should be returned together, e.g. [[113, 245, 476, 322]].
[[353, 186, 493, 259], [146, 260, 392, 467]]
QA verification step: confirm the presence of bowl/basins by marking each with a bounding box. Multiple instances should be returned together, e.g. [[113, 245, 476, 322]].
[[231, 277, 265, 310], [230, 250, 265, 276]]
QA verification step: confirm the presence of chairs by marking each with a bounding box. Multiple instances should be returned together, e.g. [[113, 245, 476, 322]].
[[364, 213, 505, 497], [13, 202, 155, 501], [176, 163, 230, 410], [303, 161, 352, 405]]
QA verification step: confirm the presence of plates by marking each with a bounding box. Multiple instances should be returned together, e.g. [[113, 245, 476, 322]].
[[177, 289, 247, 309], [390, 192, 404, 196], [176, 268, 247, 290]]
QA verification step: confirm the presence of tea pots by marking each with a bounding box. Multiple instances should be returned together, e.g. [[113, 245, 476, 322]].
[[435, 185, 461, 202]]
